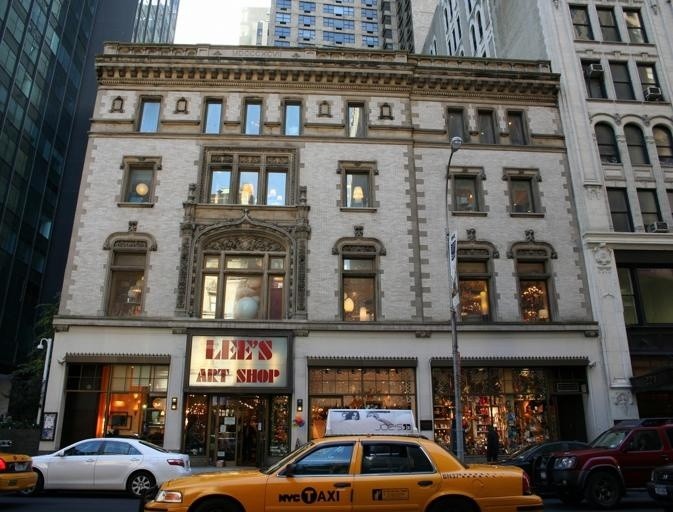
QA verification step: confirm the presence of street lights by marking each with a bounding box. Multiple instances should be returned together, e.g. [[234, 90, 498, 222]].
[[445, 136, 464, 463], [35, 336, 52, 429]]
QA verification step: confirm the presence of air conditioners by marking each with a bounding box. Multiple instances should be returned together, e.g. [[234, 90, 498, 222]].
[[649, 221, 670, 234], [644, 87, 661, 100], [587, 64, 604, 79]]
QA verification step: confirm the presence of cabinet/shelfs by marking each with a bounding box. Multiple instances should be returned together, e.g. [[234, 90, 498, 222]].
[[432, 366, 550, 455]]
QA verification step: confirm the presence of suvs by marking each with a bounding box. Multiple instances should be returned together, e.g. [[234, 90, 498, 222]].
[[532, 417, 672, 510]]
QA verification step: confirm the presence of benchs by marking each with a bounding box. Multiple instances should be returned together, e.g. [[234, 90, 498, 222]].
[[395, 463, 415, 472]]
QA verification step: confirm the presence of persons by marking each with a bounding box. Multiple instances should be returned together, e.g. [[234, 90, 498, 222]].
[[110, 424, 120, 435], [316, 445, 351, 474], [485, 427, 498, 463], [143, 422, 148, 434], [358, 300, 373, 321], [635, 433, 652, 451], [190, 413, 199, 433], [191, 423, 205, 455], [343, 411, 358, 421], [242, 420, 256, 462], [312, 411, 326, 422], [407, 402, 410, 409], [544, 426, 549, 440], [505, 399, 511, 409], [237, 415, 243, 426], [354, 402, 381, 409]]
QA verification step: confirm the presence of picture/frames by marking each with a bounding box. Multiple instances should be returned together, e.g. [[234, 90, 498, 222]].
[[40, 412, 58, 440]]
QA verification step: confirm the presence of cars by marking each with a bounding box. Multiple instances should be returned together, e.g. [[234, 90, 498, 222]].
[[139, 408, 543, 511], [14, 437, 191, 498], [475, 441, 608, 504], [0, 451, 37, 494], [643, 461, 672, 508], [0, 439, 16, 452]]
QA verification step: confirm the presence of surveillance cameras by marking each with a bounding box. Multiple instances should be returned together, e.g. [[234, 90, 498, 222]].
[[589, 360, 596, 367], [57, 358, 64, 365], [37, 344, 44, 352]]
